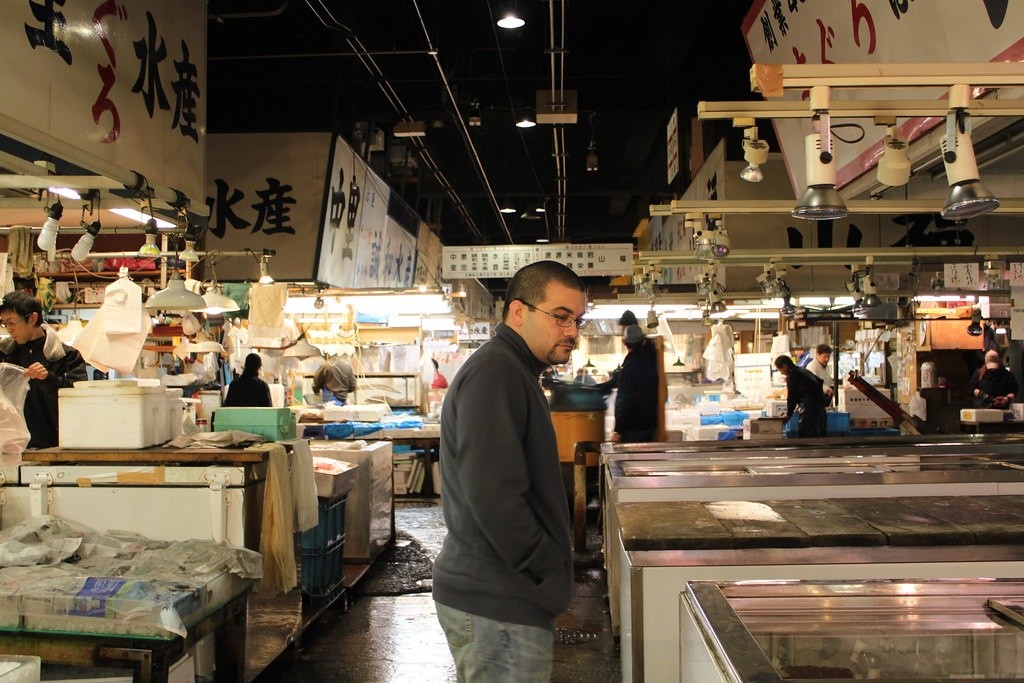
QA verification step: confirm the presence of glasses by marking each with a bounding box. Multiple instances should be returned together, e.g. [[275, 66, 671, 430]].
[[1, 313, 31, 331], [514, 298, 590, 330]]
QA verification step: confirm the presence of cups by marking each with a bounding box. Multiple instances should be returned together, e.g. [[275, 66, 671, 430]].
[[938, 377, 946, 385], [1011, 404, 1024, 419]]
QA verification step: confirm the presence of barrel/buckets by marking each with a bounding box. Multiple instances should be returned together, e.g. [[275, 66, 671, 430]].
[[920, 361, 937, 388]]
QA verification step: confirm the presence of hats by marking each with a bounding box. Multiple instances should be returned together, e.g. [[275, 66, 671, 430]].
[[623, 324, 644, 343], [985, 349, 1000, 370]]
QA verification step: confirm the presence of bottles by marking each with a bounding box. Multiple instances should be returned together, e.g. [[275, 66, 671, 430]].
[[196, 419, 208, 432]]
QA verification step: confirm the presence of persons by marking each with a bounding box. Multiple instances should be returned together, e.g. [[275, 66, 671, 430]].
[[972, 350, 1017, 410], [775, 355, 828, 438], [0, 291, 88, 449], [795, 346, 812, 368], [432, 261, 586, 682], [313, 352, 356, 406], [223, 353, 273, 406], [576, 368, 599, 384], [597, 311, 659, 443], [431, 358, 447, 389], [806, 345, 835, 406]]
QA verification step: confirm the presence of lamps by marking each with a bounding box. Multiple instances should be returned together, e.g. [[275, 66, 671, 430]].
[[1, 150, 326, 312], [633, 58, 1024, 367], [392, 0, 599, 246]]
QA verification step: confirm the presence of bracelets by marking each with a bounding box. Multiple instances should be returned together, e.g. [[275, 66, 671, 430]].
[[1007, 396, 1011, 403]]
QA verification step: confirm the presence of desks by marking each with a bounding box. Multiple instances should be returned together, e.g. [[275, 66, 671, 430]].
[[0, 579, 256, 683], [309, 425, 440, 501]]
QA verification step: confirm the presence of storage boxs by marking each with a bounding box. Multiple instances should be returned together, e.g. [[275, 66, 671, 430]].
[[605, 321, 1024, 443], [0, 318, 448, 683]]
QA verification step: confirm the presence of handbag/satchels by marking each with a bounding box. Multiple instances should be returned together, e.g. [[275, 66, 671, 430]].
[[782, 412, 803, 439]]
